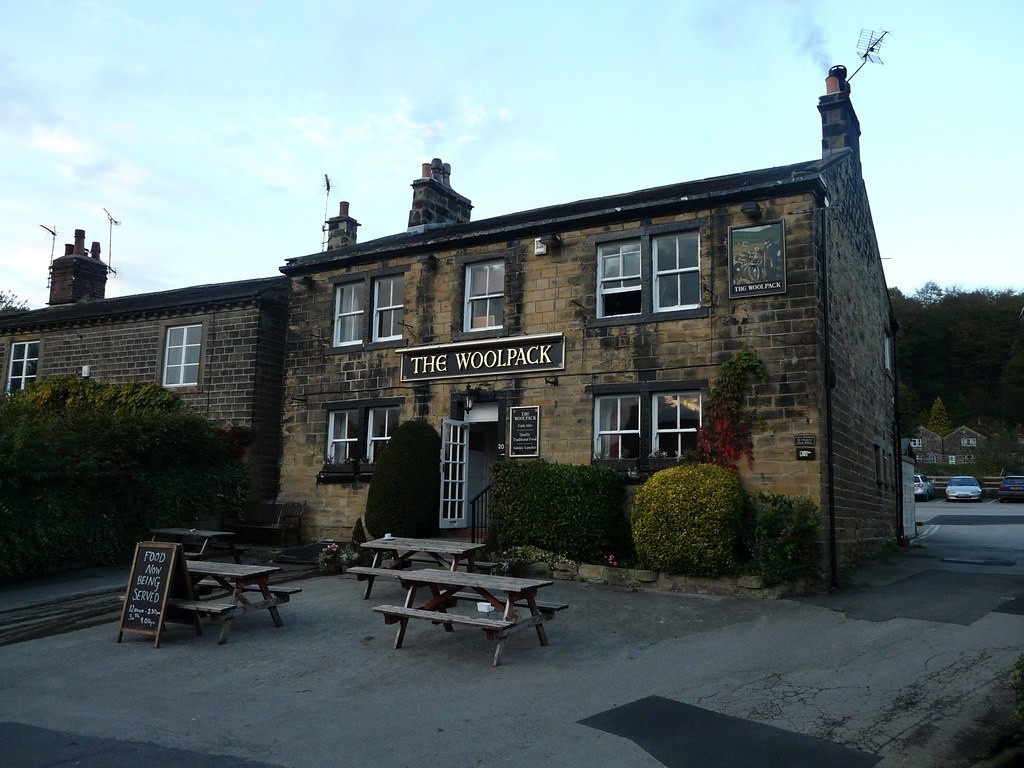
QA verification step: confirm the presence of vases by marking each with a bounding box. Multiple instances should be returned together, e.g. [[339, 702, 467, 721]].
[[341, 564, 351, 574], [327, 560, 339, 571]]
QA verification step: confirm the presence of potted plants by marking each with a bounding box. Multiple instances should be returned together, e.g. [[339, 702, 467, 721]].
[[899, 534, 910, 548]]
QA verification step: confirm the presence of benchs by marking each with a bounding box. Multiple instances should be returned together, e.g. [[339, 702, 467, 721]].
[[372, 605, 517, 631], [168, 597, 237, 614], [185, 553, 206, 558], [187, 543, 252, 551], [391, 555, 500, 569], [439, 590, 570, 611], [218, 500, 308, 546], [345, 567, 407, 578], [196, 580, 303, 594]]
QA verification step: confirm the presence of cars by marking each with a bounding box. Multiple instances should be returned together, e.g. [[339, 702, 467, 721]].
[[945, 476, 983, 503], [998, 476, 1024, 503]]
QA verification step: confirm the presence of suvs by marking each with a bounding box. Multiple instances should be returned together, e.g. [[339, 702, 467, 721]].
[[914, 473, 937, 502]]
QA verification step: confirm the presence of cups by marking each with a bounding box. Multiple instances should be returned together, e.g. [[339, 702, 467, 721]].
[[385, 534, 391, 539]]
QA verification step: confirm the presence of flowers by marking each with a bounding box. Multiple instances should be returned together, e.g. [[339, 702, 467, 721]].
[[316, 543, 359, 574]]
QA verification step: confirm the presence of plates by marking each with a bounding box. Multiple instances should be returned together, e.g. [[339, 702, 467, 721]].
[[381, 537, 394, 540]]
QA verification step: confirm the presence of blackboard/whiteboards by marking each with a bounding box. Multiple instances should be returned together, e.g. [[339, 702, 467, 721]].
[[509, 406, 539, 457], [121, 543, 176, 634]]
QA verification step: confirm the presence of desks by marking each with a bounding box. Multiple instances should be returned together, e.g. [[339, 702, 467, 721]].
[[392, 569, 554, 665], [360, 536, 486, 601], [150, 528, 242, 564], [185, 560, 283, 643]]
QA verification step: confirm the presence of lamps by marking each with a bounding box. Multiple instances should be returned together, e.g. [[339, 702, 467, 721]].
[[460, 381, 478, 413], [539, 234, 564, 249], [739, 201, 764, 218], [418, 253, 440, 266], [294, 275, 315, 287]]
